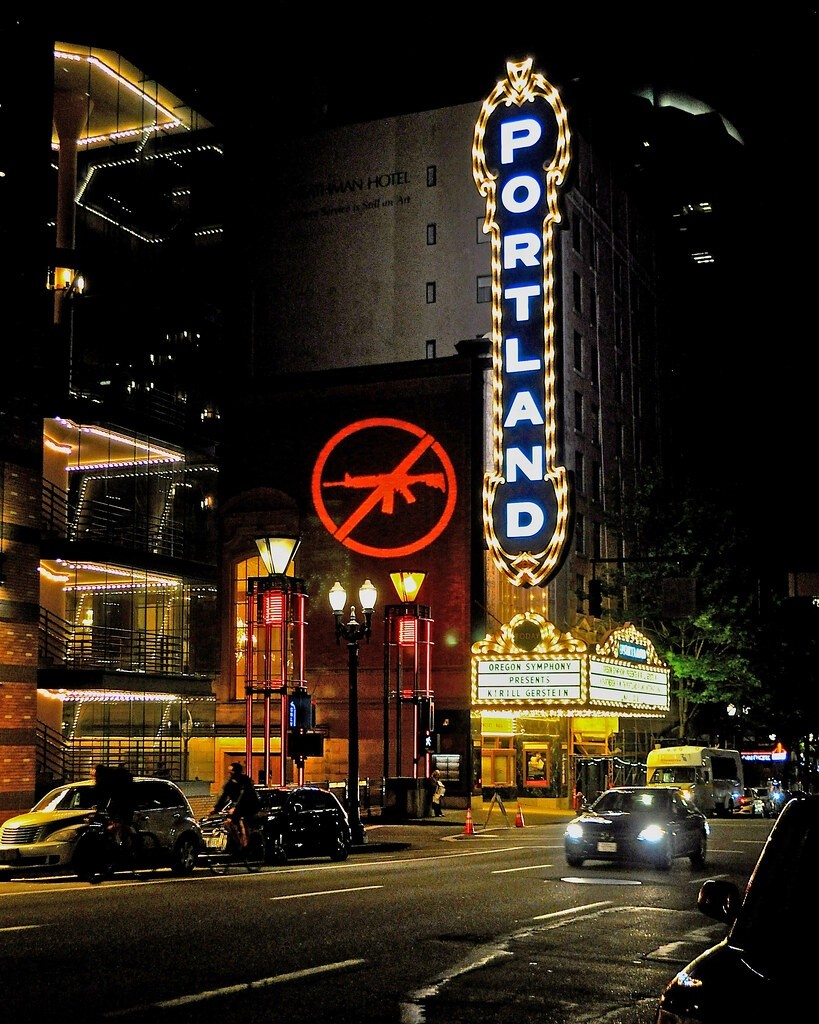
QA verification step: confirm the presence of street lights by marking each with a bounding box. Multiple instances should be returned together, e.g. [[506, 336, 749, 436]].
[[382, 569, 436, 779], [328, 580, 377, 845], [727, 702, 752, 751], [244, 535, 309, 785]]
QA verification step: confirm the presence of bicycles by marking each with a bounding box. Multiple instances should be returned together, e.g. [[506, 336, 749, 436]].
[[65, 811, 163, 884], [211, 810, 265, 876]]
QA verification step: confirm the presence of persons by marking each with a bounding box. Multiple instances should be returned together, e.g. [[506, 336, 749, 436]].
[[430, 769, 445, 817], [94, 765, 135, 842], [208, 763, 262, 833]]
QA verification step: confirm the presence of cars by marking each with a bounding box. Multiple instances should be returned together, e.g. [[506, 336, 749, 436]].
[[732, 787, 808, 819], [565, 787, 709, 869], [201, 787, 353, 866], [0, 776, 200, 879], [651, 799, 819, 1024]]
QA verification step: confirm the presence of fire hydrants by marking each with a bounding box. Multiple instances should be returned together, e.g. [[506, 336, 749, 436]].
[[575, 792, 583, 816]]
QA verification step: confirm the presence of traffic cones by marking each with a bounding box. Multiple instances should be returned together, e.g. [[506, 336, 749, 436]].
[[461, 808, 478, 834], [514, 803, 525, 829]]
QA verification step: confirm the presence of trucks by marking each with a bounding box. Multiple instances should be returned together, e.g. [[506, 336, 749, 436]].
[[646, 745, 745, 818]]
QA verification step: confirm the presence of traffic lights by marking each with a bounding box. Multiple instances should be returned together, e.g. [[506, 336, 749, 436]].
[[424, 736, 436, 752]]
